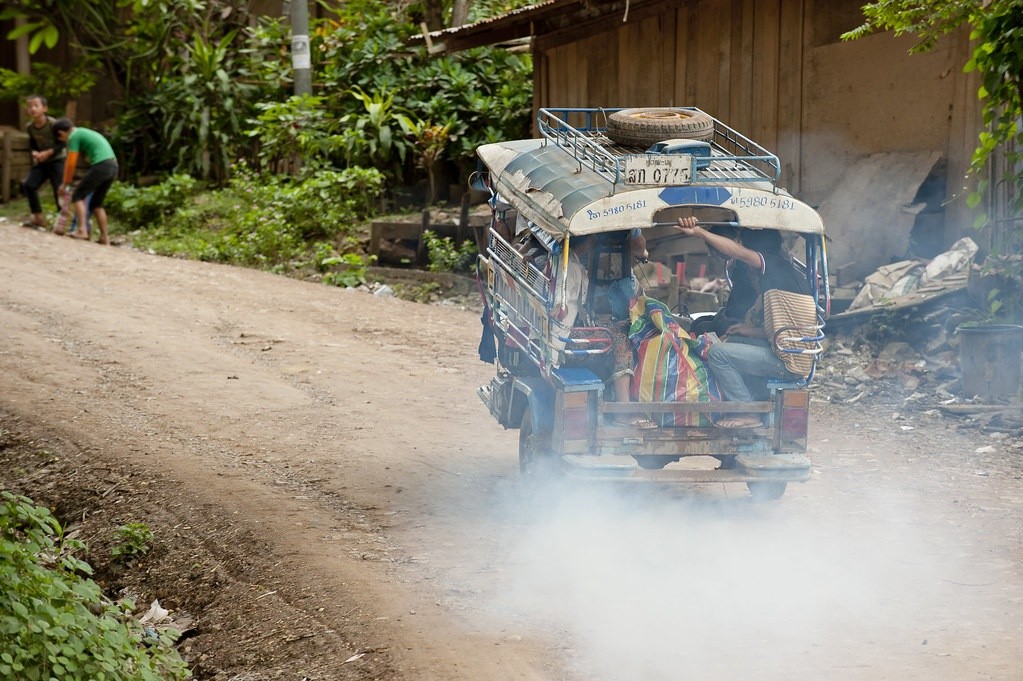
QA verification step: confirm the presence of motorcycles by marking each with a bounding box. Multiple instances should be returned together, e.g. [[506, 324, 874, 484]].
[[467, 107, 832, 501]]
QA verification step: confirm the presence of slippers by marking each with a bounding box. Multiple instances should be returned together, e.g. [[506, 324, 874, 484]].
[[612, 417, 657, 430], [66, 232, 88, 240], [23, 218, 47, 228], [714, 416, 762, 429]]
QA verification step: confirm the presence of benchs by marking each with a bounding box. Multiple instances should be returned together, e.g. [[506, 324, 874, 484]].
[[485, 227, 605, 392], [761, 318, 828, 388]]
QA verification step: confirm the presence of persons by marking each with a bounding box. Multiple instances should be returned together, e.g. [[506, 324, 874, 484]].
[[20, 95, 66, 230], [52, 118, 118, 246], [672, 217, 818, 430], [488, 193, 658, 429]]
